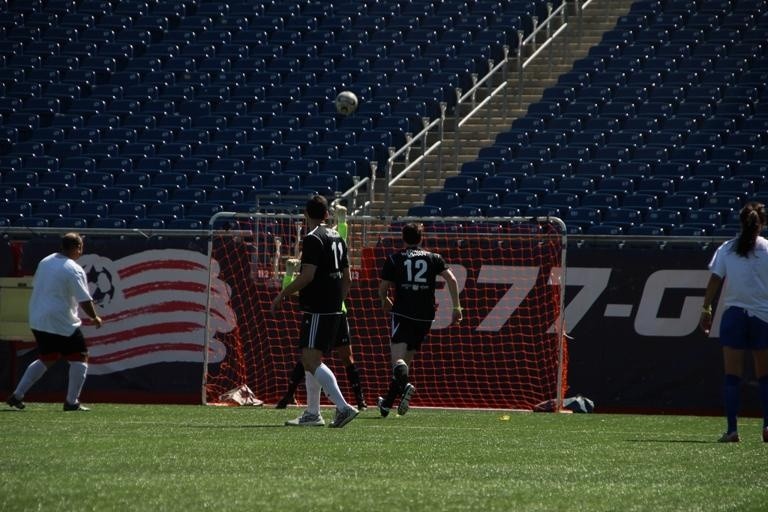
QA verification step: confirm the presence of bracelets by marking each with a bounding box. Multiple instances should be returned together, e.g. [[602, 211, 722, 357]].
[[451, 305, 466, 312], [701, 306, 713, 318]]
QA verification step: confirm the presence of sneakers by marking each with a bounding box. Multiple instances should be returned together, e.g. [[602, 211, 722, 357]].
[[277, 394, 295, 408], [64, 399, 90, 411], [358, 399, 367, 412], [717, 430, 740, 442], [286, 410, 325, 426], [329, 404, 360, 427], [6, 393, 26, 409], [763, 426, 768, 441], [398, 382, 416, 415], [376, 396, 390, 417]]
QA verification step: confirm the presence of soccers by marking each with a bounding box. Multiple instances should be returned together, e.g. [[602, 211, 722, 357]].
[[336, 91, 359, 114], [80, 264, 115, 309]]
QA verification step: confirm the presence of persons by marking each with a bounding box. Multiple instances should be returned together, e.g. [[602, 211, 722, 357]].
[[374, 220, 466, 418], [274, 201, 368, 411], [7, 230, 102, 412], [696, 202, 768, 447], [269, 195, 361, 429]]
